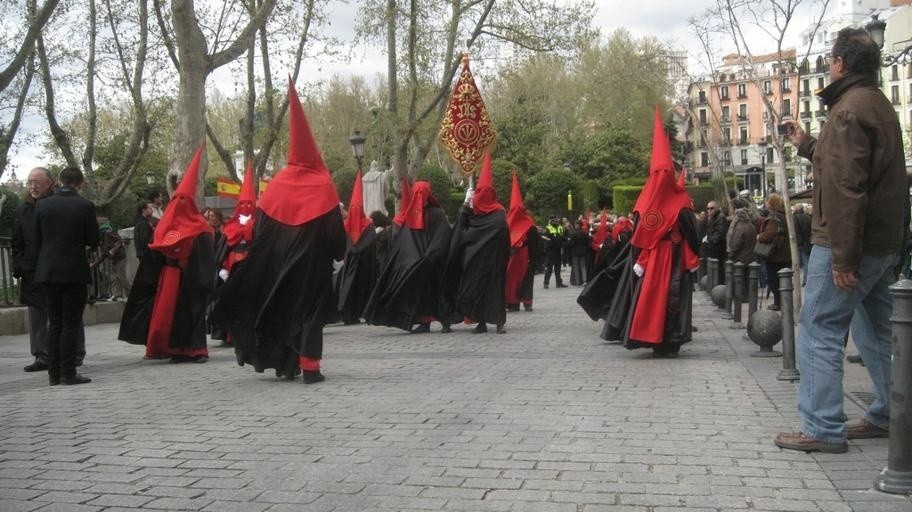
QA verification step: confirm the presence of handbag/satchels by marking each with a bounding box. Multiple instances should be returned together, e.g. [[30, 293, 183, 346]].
[[753, 239, 776, 258]]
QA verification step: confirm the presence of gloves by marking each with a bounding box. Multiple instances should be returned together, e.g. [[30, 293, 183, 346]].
[[332, 259, 345, 275], [219, 269, 228, 281], [464, 187, 476, 206], [238, 214, 251, 225], [633, 263, 645, 277]]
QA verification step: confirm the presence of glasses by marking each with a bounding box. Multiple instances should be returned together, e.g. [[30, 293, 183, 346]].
[[707, 207, 714, 210]]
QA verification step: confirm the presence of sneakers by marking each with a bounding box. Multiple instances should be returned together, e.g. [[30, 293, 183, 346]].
[[441, 323, 451, 333], [473, 325, 487, 333], [847, 355, 865, 365], [846, 418, 889, 439], [653, 351, 678, 358], [525, 306, 533, 311], [497, 325, 506, 334], [304, 368, 325, 384], [410, 322, 430, 333], [775, 431, 848, 453]]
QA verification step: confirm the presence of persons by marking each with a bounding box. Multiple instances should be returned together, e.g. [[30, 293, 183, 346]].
[[10, 157, 547, 384], [776, 25, 908, 454], [533, 158, 814, 357]]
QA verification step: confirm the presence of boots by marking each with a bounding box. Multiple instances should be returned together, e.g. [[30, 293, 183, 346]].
[[544, 282, 549, 288], [556, 277, 568, 288]]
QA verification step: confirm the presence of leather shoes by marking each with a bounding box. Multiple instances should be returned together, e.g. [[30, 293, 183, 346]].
[[48, 365, 92, 386], [24, 360, 49, 372]]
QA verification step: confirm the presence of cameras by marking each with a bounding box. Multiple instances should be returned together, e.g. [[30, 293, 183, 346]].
[[775, 122, 793, 137]]
